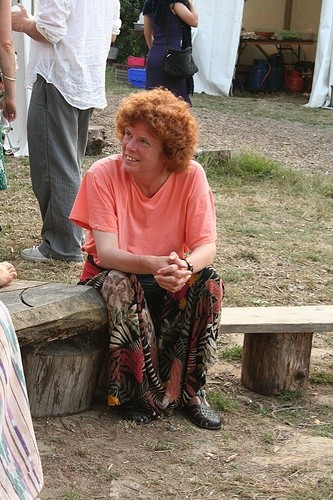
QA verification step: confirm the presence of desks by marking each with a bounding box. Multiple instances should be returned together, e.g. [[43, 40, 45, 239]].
[[240, 38, 316, 95]]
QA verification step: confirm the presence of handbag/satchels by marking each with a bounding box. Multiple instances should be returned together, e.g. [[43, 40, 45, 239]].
[[164, 49, 198, 78]]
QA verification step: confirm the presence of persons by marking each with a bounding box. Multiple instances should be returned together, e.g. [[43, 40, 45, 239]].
[[11, 1, 122, 262], [68, 86, 225, 432], [143, 0, 198, 106], [0, 1, 18, 287]]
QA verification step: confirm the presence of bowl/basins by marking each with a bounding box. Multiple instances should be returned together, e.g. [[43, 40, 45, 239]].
[[300, 32, 315, 41], [255, 32, 275, 39]]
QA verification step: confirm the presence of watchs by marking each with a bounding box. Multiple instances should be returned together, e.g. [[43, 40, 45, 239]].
[[181, 259, 194, 273]]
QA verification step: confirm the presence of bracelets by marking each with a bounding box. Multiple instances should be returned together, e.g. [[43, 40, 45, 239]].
[[3, 75, 17, 81]]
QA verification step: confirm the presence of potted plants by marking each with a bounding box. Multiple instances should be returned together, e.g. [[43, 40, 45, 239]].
[[113, 0, 149, 85]]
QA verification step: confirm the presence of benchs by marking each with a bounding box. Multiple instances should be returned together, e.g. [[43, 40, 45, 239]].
[[0, 278, 108, 419], [217, 305, 333, 399]]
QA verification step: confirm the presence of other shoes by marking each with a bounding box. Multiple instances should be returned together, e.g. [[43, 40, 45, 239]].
[[21, 246, 58, 263], [186, 391, 222, 430]]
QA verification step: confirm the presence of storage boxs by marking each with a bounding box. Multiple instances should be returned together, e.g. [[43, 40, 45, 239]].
[[127, 55, 146, 67], [127, 67, 147, 89]]
